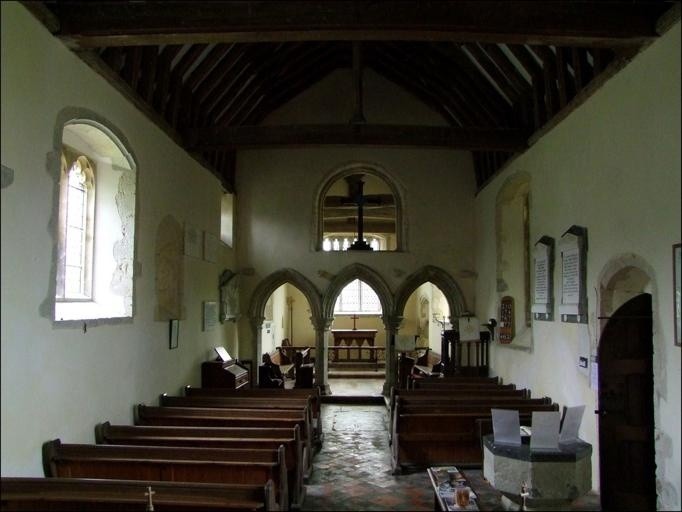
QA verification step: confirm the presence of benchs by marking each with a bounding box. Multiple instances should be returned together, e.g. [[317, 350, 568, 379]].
[[1, 384, 322, 512], [411, 350, 442, 376], [262, 346, 295, 381], [386, 376, 559, 478]]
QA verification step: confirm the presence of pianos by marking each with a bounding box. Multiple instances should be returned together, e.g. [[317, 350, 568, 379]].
[[201, 361, 250, 390]]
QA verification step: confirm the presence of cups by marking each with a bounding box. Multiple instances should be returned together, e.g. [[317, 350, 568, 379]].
[[455, 486, 470, 508]]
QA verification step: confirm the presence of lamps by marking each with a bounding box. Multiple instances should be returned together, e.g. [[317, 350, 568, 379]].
[[479, 316, 496, 336]]
